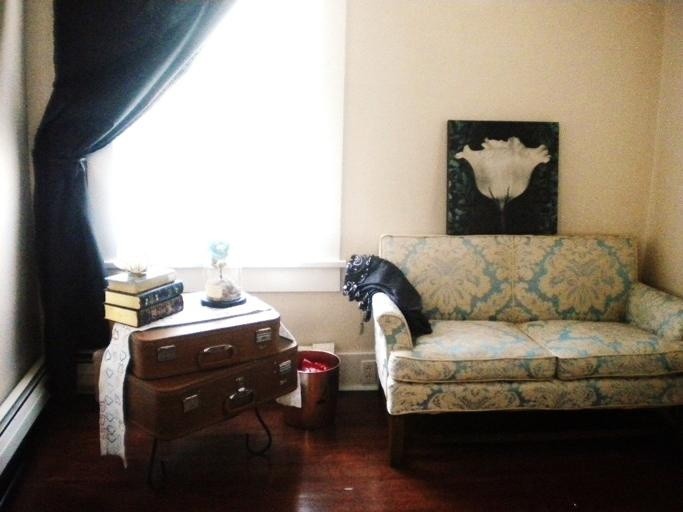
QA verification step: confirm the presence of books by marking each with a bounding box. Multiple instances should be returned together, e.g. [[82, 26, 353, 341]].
[[103, 271, 184, 327]]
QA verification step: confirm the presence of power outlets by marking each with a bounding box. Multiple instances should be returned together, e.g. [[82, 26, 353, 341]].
[[360, 360, 377, 385]]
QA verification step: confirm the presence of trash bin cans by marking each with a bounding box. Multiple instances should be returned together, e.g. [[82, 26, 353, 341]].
[[282, 350, 341, 430]]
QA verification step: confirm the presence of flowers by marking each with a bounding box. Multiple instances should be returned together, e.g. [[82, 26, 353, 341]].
[[454, 136, 552, 235]]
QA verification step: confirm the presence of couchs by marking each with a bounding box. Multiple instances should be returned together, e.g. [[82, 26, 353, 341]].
[[372, 235, 682, 468]]
